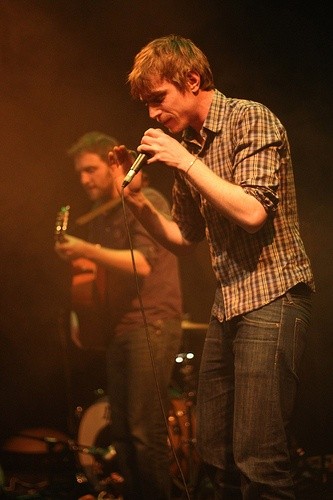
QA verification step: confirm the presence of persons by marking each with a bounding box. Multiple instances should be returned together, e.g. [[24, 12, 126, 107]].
[[55, 135, 180, 499], [125, 34, 317, 500]]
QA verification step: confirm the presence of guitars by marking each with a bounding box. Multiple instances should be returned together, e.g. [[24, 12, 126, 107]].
[[57, 205, 107, 330]]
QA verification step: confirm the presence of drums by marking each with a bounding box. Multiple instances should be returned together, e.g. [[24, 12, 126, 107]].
[[77, 386, 199, 493]]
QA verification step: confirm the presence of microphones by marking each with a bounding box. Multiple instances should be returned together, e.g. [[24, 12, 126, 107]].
[[122, 126, 167, 187]]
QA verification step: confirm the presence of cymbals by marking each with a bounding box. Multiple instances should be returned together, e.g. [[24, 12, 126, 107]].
[[179, 320, 210, 329]]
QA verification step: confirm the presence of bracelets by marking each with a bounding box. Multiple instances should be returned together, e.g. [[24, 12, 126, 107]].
[[184, 153, 201, 177]]
